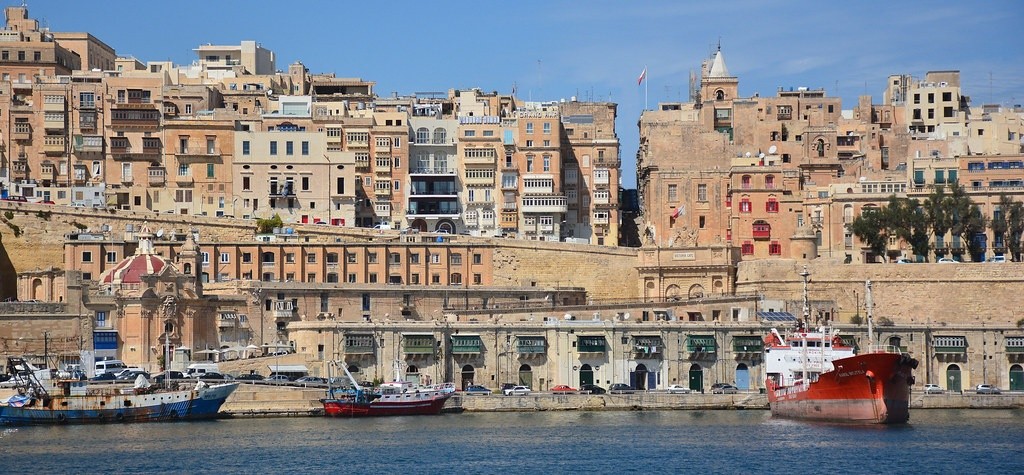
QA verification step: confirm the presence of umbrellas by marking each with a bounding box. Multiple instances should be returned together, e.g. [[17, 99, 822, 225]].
[[194, 344, 261, 361]]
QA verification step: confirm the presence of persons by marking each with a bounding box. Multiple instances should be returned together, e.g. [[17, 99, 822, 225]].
[[468, 381, 471, 386], [249, 352, 273, 358]]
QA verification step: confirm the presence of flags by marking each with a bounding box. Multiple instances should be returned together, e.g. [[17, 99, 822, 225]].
[[672, 206, 685, 219], [638, 68, 645, 85]]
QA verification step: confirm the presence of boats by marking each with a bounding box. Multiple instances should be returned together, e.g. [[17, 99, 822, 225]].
[[318, 380, 457, 416], [761, 324, 920, 425], [0, 373, 242, 422]]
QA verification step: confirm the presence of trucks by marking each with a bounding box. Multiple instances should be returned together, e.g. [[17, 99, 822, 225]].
[[373, 225, 391, 229]]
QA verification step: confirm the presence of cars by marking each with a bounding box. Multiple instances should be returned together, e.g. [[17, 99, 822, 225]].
[[550, 385, 577, 394], [294, 376, 323, 388], [501, 385, 531, 397], [464, 385, 492, 396], [976, 383, 1002, 395], [709, 382, 739, 395], [669, 385, 690, 394], [922, 383, 945, 394], [580, 384, 606, 395], [0, 195, 86, 208], [610, 383, 634, 395], [263, 374, 291, 386], [896, 256, 1006, 264], [235, 374, 263, 384], [0, 359, 235, 388]]
